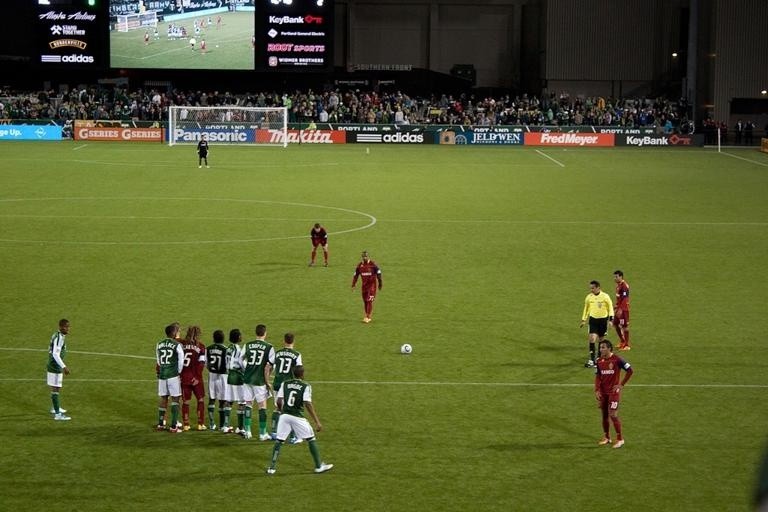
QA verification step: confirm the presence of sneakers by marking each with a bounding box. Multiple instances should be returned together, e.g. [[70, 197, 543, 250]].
[[210, 423, 253, 439], [315, 463, 334, 473], [197, 424, 207, 431], [599, 438, 611, 446], [267, 467, 275, 474], [197, 165, 211, 168], [260, 432, 277, 441], [612, 439, 625, 448], [362, 316, 370, 323], [586, 360, 594, 367], [615, 341, 632, 350], [289, 437, 302, 444], [156, 421, 192, 433], [309, 261, 328, 267], [50, 405, 72, 421]]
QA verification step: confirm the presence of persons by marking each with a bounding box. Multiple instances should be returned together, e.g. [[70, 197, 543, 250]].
[[197, 135, 210, 169], [143, 15, 222, 56], [46, 318, 74, 421], [611, 269, 632, 350], [153, 323, 305, 443], [595, 340, 633, 449], [309, 220, 330, 269], [268, 368, 337, 473], [0, 88, 694, 143], [574, 280, 617, 369], [352, 250, 384, 323], [700, 117, 756, 145]]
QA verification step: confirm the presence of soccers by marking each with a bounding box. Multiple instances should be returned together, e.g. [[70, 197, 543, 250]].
[[401, 343, 412, 353], [215, 45, 219, 48]]
